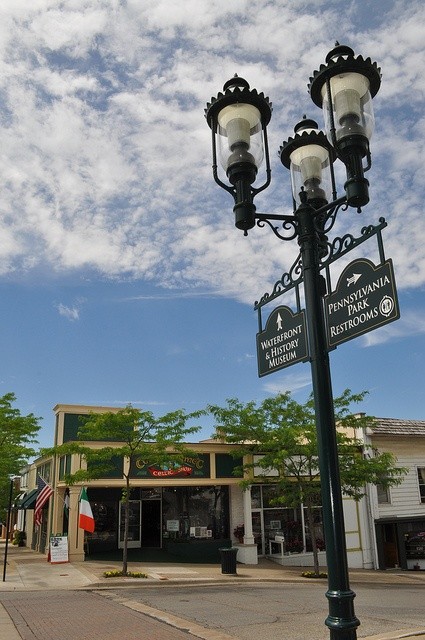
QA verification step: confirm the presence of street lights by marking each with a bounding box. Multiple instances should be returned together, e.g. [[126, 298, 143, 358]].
[[203, 40, 381, 640]]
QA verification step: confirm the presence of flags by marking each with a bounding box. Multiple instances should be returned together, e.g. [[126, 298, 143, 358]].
[[34, 475, 54, 526], [64, 489, 69, 520], [78, 487, 95, 534]]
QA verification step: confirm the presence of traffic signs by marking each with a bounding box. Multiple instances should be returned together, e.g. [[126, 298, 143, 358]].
[[323, 258, 401, 352], [256, 303, 307, 378]]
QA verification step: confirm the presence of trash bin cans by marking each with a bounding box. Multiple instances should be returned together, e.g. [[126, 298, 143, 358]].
[[219, 548, 238, 574]]
